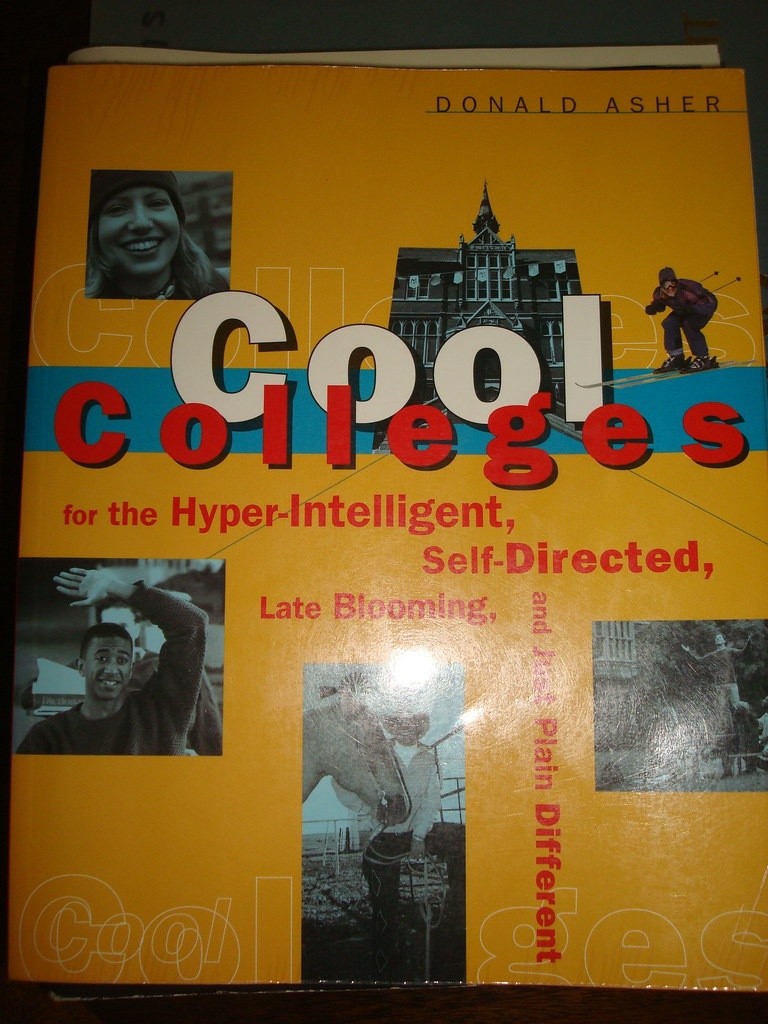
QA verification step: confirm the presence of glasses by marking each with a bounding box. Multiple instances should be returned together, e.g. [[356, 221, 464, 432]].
[[660, 280, 676, 289]]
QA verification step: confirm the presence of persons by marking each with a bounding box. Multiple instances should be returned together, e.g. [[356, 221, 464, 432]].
[[331, 695, 444, 922], [681, 631, 754, 729], [14, 568, 209, 756], [87, 170, 229, 300], [645, 266, 719, 370]]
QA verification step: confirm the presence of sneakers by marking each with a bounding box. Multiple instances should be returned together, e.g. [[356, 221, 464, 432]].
[[689, 354, 710, 368], [661, 351, 685, 369]]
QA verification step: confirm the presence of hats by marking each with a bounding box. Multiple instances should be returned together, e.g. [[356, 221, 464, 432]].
[[90, 170, 187, 224], [659, 267, 675, 282]]
